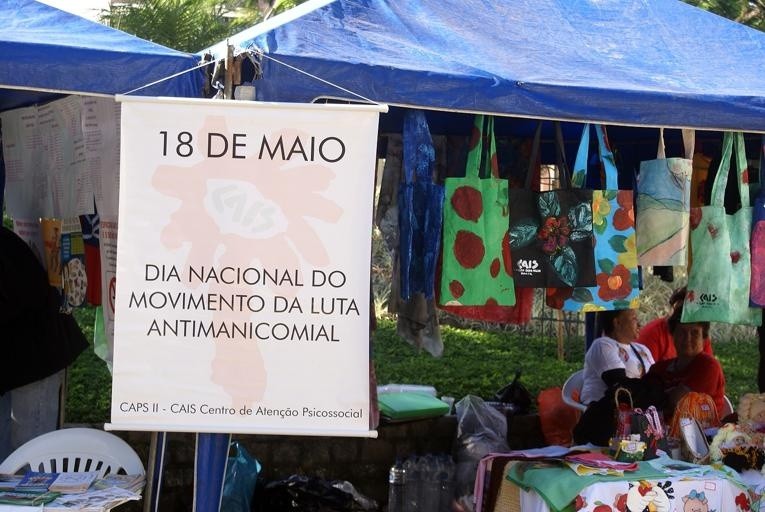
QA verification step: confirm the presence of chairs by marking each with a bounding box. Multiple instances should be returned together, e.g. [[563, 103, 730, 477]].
[[0, 428, 145, 474], [562, 369, 589, 424]]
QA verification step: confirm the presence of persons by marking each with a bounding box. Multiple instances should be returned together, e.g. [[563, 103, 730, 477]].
[[644, 303, 726, 423], [581, 310, 655, 405], [635, 284, 715, 362]]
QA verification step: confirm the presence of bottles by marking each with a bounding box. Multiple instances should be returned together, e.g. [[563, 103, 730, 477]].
[[406, 456, 421, 512], [426, 456, 441, 512], [442, 456, 456, 512], [389, 457, 406, 512]]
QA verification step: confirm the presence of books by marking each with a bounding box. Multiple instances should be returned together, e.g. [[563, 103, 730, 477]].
[[1, 471, 147, 510]]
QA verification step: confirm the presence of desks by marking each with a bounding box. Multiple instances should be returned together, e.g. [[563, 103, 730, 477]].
[[381, 414, 445, 466]]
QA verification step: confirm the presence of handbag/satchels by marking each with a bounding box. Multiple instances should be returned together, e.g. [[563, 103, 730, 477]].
[[680, 132, 753, 324], [439, 114, 515, 307], [546, 125, 641, 313], [637, 125, 696, 268], [510, 120, 597, 290]]
